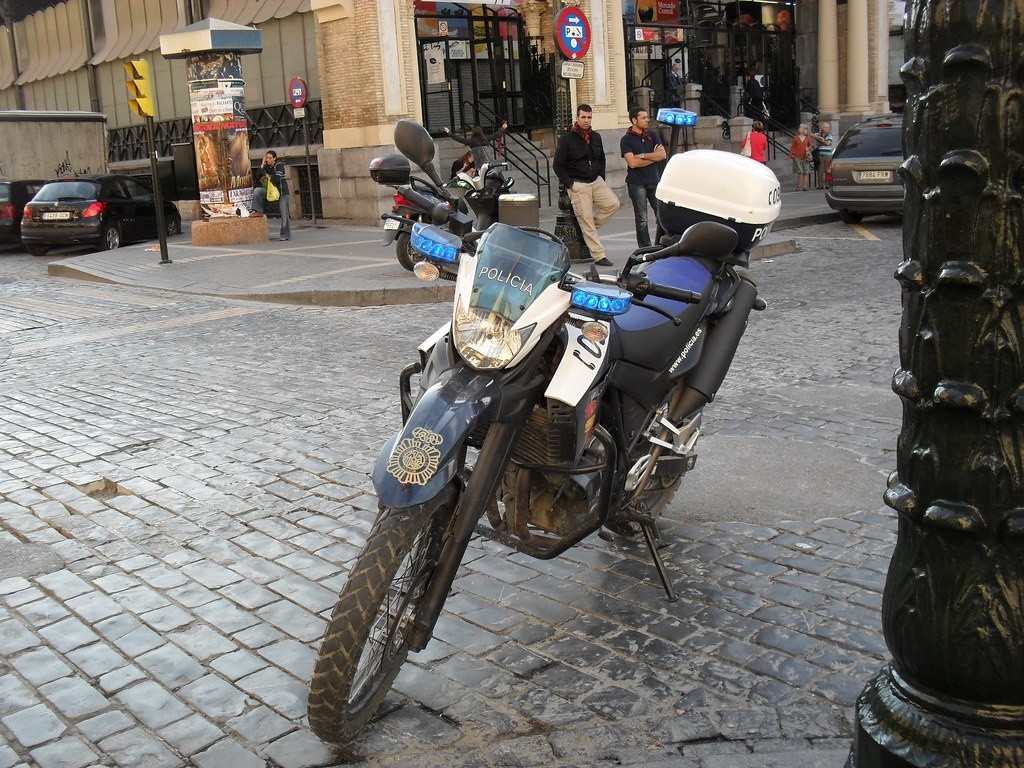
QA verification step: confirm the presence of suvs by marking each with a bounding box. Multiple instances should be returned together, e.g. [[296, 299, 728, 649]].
[[823, 112, 907, 224]]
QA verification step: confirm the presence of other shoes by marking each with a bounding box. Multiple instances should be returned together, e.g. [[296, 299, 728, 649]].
[[816, 186, 823, 190], [803, 188, 808, 191], [826, 187, 828, 189], [796, 188, 799, 191], [595, 258, 613, 266], [280, 238, 285, 241], [250, 212, 263, 217]]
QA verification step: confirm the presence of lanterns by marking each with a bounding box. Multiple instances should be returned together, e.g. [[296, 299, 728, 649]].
[[761, 3, 777, 32]]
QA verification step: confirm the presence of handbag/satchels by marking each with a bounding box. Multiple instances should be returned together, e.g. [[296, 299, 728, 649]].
[[812, 147, 821, 171], [267, 176, 280, 201], [806, 136, 813, 162], [236, 202, 249, 217], [741, 132, 751, 157]]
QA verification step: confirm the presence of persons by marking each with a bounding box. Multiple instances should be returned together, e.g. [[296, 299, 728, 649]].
[[743, 63, 770, 121], [553, 103, 614, 266], [620, 107, 667, 251], [788, 122, 833, 191], [665, 61, 689, 107], [250, 150, 290, 242], [738, 120, 769, 165], [444, 121, 508, 185]]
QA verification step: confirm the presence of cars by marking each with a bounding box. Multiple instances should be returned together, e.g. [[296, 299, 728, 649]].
[[0, 179, 48, 244], [21, 173, 181, 256]]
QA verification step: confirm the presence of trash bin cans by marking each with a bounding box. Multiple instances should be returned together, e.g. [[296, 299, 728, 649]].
[[498, 194, 539, 236]]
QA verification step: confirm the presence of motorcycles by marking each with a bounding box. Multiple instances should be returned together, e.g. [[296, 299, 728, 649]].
[[306, 121, 782, 746], [369, 152, 516, 272]]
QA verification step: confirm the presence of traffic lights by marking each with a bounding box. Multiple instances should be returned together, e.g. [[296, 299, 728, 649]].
[[124, 57, 154, 119]]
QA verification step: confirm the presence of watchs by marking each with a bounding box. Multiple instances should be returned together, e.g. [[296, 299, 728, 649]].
[[640, 153, 644, 160]]
